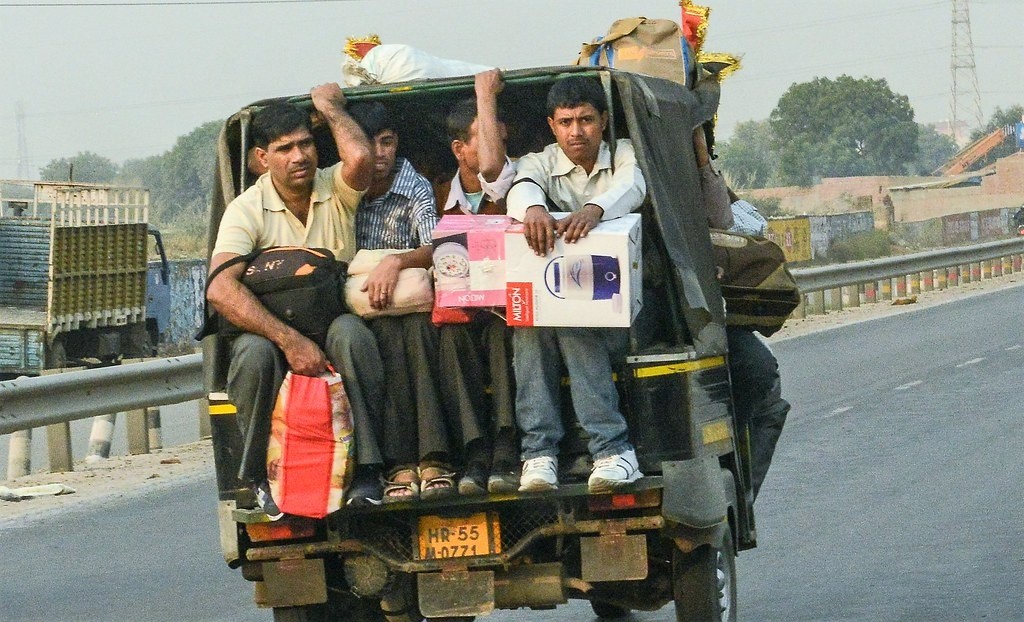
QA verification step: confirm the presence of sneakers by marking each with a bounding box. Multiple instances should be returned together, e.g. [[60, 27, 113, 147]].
[[255, 482, 287, 522], [587, 449, 643, 496], [459, 464, 487, 494], [518, 455, 558, 492], [344, 474, 382, 506], [488, 462, 520, 492]]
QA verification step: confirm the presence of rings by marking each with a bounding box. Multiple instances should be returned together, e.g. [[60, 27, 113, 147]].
[[381, 291, 388, 293]]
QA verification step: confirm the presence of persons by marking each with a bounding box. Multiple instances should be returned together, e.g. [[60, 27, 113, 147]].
[[430, 67, 515, 496], [206, 82, 382, 521], [709, 186, 791, 503], [509, 77, 648, 492], [247, 101, 454, 503]]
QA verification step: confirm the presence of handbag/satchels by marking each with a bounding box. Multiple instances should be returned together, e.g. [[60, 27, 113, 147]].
[[706, 227, 801, 329], [267, 362, 355, 520], [345, 248, 433, 321], [218, 246, 349, 335], [574, 17, 695, 89]]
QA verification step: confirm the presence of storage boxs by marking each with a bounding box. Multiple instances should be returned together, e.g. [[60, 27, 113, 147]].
[[432, 214, 512, 306], [503, 212, 644, 327]]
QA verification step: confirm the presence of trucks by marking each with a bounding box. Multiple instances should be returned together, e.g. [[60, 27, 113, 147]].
[[0, 179, 172, 377], [206, 67, 756, 622]]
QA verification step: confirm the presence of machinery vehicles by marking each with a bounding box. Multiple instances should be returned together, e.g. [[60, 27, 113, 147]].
[[930, 129, 1007, 178]]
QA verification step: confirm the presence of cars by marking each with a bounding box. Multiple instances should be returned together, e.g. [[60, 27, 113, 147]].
[[1017, 225, 1024, 235]]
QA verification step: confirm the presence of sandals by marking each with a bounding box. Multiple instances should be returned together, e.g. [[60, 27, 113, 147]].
[[378, 462, 417, 504], [419, 466, 456, 500]]
